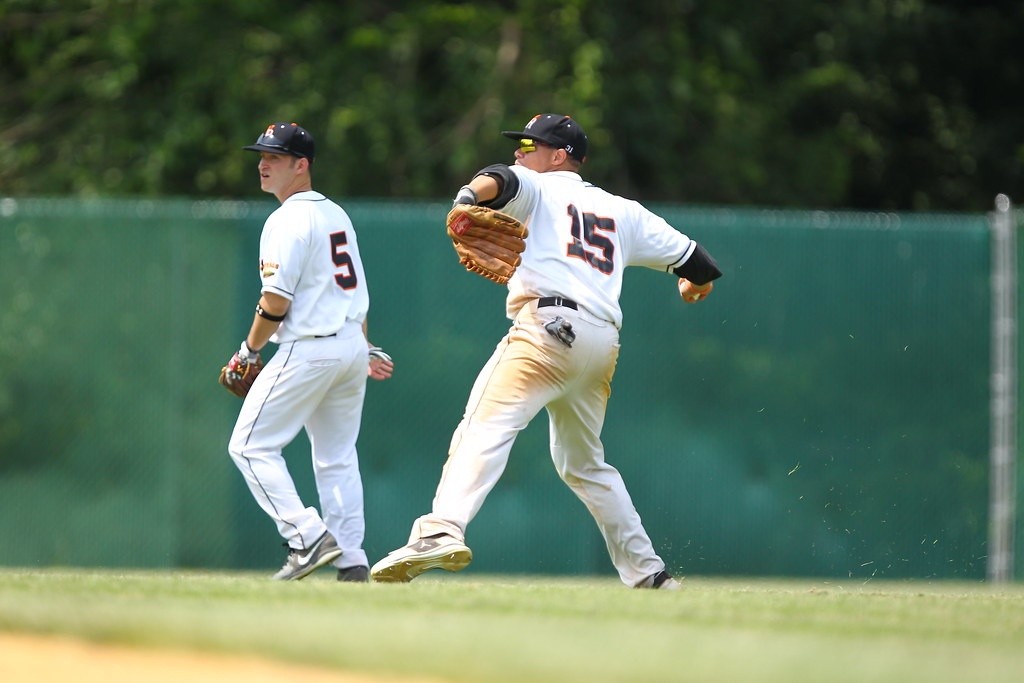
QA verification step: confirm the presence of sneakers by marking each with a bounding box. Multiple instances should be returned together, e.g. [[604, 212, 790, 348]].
[[337, 561, 368, 582], [367, 532, 475, 583], [632, 569, 680, 590], [272, 530, 343, 582]]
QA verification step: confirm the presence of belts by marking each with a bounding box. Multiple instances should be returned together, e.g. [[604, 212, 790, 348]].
[[539, 296, 577, 309]]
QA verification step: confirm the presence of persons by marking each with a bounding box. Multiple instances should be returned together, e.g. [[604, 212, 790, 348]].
[[218, 123, 393, 581], [369, 113, 723, 590]]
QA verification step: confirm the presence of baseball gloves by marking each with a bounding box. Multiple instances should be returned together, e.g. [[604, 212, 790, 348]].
[[446, 203, 529, 285], [221, 353, 264, 398]]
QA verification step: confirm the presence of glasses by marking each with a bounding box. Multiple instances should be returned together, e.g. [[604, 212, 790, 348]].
[[518, 138, 536, 154]]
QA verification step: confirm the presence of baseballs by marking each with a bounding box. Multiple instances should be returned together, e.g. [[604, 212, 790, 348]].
[[679, 282, 701, 301]]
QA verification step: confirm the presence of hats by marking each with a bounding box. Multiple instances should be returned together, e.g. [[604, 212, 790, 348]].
[[501, 113, 587, 165], [241, 122, 316, 165]]
